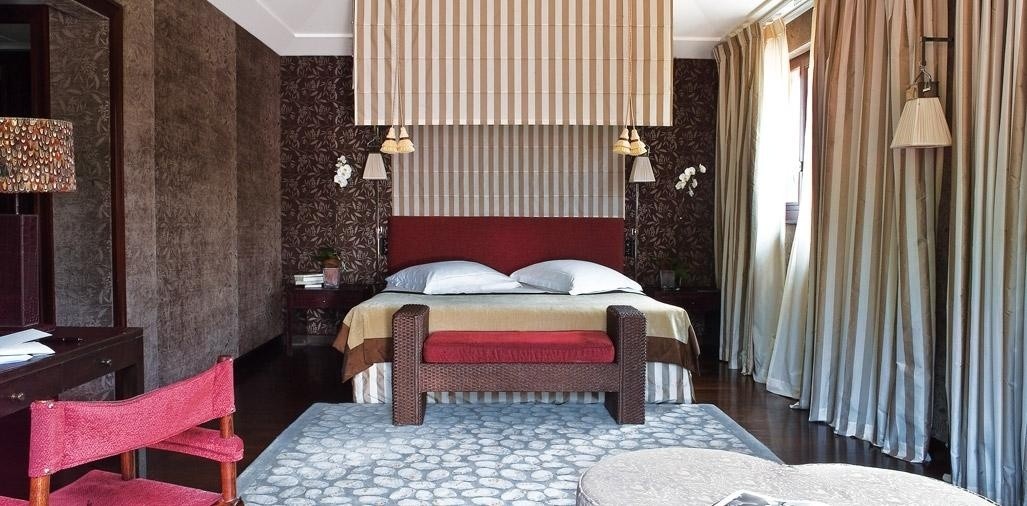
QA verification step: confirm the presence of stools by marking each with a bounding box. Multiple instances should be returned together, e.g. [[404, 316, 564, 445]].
[[576, 445, 1001, 506]]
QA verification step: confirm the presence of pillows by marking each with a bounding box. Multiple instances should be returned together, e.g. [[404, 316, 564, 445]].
[[504, 256, 644, 298], [386, 254, 518, 298]]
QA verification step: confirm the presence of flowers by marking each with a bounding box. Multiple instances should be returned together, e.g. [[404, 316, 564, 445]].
[[671, 164, 707, 201], [327, 155, 353, 190]]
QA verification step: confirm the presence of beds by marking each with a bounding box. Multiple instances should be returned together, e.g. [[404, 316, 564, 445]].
[[334, 207, 702, 426]]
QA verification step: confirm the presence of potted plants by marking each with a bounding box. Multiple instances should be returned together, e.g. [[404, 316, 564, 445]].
[[313, 247, 341, 288], [650, 250, 688, 291]]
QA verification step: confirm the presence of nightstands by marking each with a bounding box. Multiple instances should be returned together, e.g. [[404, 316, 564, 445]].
[[283, 281, 370, 349], [637, 288, 720, 373]]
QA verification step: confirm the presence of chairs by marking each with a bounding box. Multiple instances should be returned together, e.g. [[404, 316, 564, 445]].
[[0, 357, 245, 505]]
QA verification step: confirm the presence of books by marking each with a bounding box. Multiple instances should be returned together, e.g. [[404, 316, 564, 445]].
[[292, 273, 324, 288]]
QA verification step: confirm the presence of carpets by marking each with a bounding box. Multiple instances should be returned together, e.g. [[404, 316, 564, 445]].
[[216, 398, 792, 506]]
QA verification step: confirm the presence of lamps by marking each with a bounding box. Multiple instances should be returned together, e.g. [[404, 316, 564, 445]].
[[627, 155, 654, 291], [890, 35, 955, 148], [0, 116, 79, 344], [361, 154, 386, 295]]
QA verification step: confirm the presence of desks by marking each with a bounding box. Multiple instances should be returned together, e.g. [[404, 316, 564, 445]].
[[0, 322, 145, 492]]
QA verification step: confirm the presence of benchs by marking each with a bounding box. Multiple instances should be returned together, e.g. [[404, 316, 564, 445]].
[[390, 302, 648, 427]]
